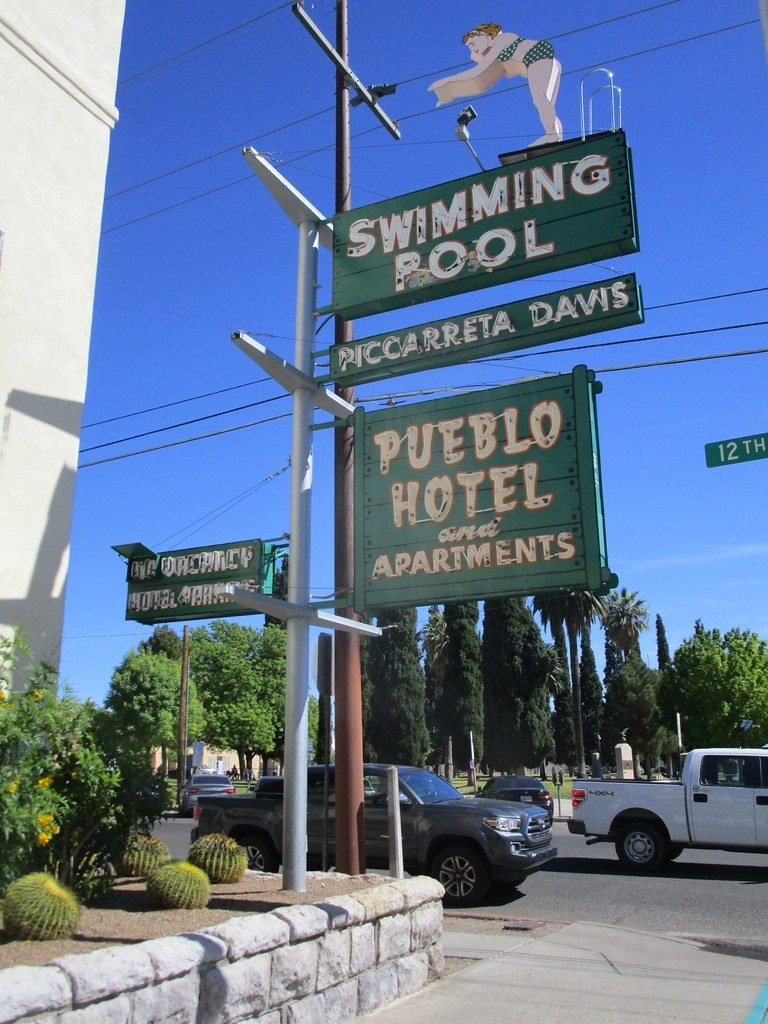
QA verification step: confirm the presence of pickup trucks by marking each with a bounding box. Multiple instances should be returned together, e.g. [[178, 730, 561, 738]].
[[191, 765, 557, 909], [567, 748, 768, 876]]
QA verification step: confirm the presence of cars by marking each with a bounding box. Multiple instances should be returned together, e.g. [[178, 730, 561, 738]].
[[180, 774, 234, 816], [194, 765, 217, 775], [473, 777, 555, 822]]
